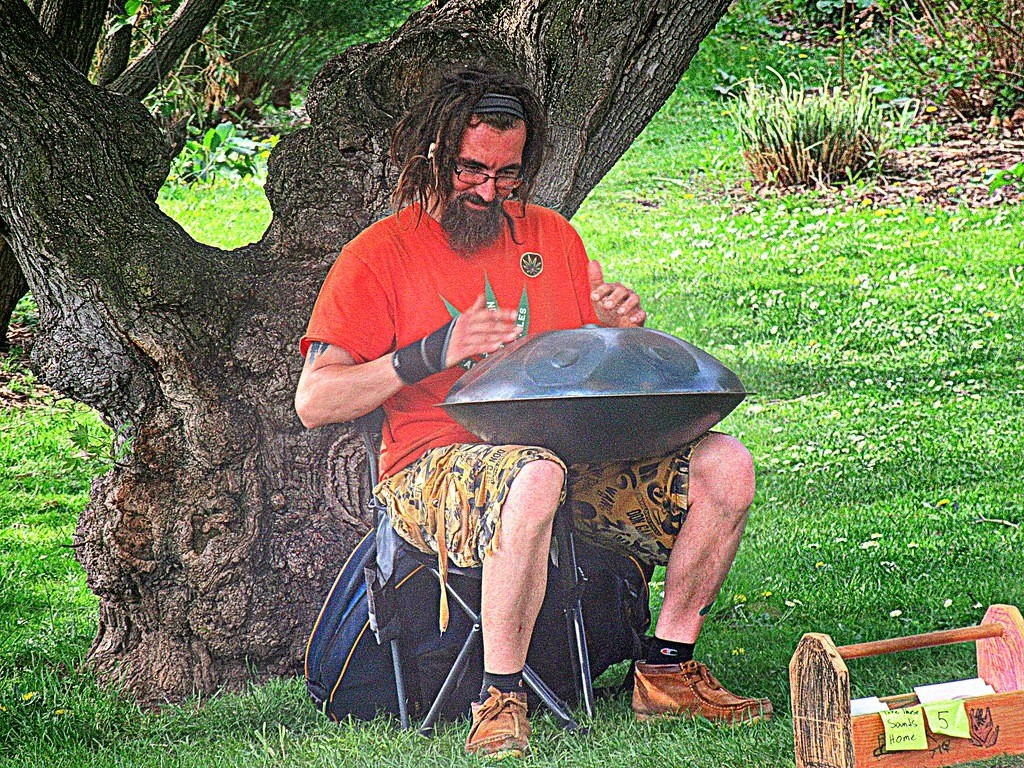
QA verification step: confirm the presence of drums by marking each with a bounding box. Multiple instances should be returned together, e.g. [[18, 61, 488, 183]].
[[433, 323, 757, 464]]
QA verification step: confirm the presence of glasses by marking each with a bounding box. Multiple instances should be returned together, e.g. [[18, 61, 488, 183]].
[[451, 159, 522, 189]]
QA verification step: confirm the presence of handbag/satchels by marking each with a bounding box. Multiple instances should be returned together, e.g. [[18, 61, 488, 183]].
[[306, 519, 653, 730]]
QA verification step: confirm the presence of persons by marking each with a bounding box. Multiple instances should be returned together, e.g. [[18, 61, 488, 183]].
[[293, 65, 772, 762]]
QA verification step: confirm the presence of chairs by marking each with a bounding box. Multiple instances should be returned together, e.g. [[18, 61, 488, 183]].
[[355, 406, 657, 737]]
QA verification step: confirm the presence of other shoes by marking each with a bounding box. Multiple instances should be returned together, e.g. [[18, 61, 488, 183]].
[[632, 660, 773, 730], [465, 692, 530, 763]]
